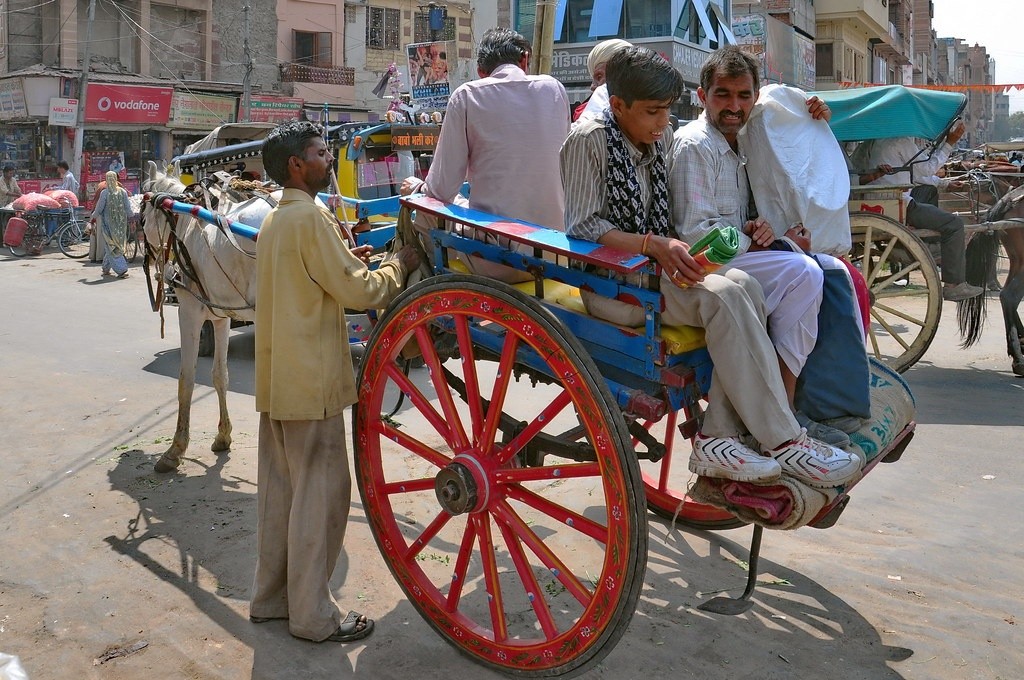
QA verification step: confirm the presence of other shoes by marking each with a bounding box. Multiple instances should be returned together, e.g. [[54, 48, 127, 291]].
[[814, 415, 860, 434], [789, 410, 850, 448]]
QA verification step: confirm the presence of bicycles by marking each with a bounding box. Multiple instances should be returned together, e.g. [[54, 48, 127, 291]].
[[1, 195, 93, 260]]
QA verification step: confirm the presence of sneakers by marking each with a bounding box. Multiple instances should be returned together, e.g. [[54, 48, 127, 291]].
[[760, 428, 863, 488], [942, 281, 982, 300], [687, 433, 781, 483]]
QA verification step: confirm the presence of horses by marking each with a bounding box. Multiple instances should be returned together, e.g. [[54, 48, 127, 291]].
[[953, 183, 1024, 378], [140, 159, 337, 475]]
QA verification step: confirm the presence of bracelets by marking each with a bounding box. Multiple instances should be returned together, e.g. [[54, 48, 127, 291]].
[[643, 230, 653, 254]]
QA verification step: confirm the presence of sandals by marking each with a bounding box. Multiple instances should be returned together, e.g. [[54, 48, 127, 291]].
[[323, 610, 375, 641]]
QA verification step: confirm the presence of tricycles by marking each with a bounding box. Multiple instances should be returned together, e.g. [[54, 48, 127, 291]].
[[123, 212, 148, 263]]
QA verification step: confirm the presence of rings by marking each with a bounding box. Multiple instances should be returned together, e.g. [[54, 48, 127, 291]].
[[672, 271, 679, 279], [680, 283, 688, 289]]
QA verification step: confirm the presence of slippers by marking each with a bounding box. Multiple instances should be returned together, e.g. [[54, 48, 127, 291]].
[[118, 272, 129, 278], [101, 272, 111, 277]]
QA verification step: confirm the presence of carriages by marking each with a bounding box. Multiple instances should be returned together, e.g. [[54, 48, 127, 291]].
[[795, 81, 1024, 384], [138, 105, 921, 680]]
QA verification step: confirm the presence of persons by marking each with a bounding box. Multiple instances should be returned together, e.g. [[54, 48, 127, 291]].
[[109, 158, 122, 175], [1009, 152, 1024, 167], [0, 165, 23, 248], [397, 26, 572, 286], [410, 48, 447, 86], [228, 162, 262, 183], [968, 153, 995, 161], [558, 38, 872, 487], [88, 170, 135, 278], [839, 122, 985, 301], [85, 141, 96, 151], [249, 121, 422, 642], [44, 159, 80, 247]]
